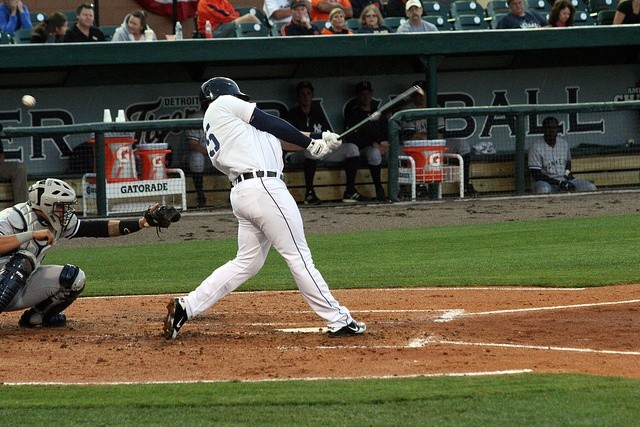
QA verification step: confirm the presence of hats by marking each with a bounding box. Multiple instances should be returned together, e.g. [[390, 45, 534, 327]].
[[291, 0, 305, 8], [405, 0, 421, 11], [296, 81, 314, 93], [356, 82, 373, 97]]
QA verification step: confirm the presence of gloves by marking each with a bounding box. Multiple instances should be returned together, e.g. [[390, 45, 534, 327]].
[[321, 130, 342, 150], [306, 138, 332, 161]]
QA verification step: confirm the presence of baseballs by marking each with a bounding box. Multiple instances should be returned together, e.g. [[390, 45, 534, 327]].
[[22, 95, 35, 107]]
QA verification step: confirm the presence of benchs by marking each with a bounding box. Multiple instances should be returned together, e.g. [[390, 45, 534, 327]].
[[450, 158, 639, 196], [1, 153, 388, 214]]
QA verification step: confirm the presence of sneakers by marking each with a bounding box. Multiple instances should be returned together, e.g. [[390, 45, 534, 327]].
[[328, 319, 366, 338], [164, 297, 188, 341], [19, 310, 66, 328], [303, 190, 321, 205], [342, 189, 369, 203]]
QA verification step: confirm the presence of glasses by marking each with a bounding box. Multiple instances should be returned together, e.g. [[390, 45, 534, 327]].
[[366, 14, 378, 18]]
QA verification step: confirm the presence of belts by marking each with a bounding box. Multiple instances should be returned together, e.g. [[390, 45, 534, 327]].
[[230, 171, 285, 190]]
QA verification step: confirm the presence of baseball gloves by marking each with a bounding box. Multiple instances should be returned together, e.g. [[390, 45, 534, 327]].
[[144, 202, 182, 239]]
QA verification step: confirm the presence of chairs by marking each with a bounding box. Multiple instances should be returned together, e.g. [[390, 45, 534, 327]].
[[21, 10, 125, 43]]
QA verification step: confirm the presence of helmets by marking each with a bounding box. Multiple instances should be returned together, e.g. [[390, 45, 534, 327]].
[[27, 178, 78, 232], [199, 77, 250, 103]]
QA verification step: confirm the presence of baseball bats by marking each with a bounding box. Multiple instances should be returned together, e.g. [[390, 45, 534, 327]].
[[337, 84, 425, 140]]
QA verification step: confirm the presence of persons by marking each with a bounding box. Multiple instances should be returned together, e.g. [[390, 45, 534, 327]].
[[30, 11, 69, 42], [528, 117, 598, 194], [395, 0, 440, 33], [180, 90, 212, 208], [0, 28, 16, 44], [285, 0, 314, 35], [356, 4, 395, 33], [197, 0, 261, 37], [612, 0, 640, 24], [351, 0, 384, 17], [401, 79, 481, 200], [345, 81, 411, 203], [110, 10, 157, 41], [0, 177, 167, 327], [263, 0, 310, 26], [64, 3, 106, 41], [163, 77, 368, 338], [311, 0, 354, 19], [280, 80, 370, 204], [0, 0, 32, 36], [496, 0, 552, 29], [548, 0, 575, 26], [318, 7, 353, 34], [387, 0, 427, 17]]
